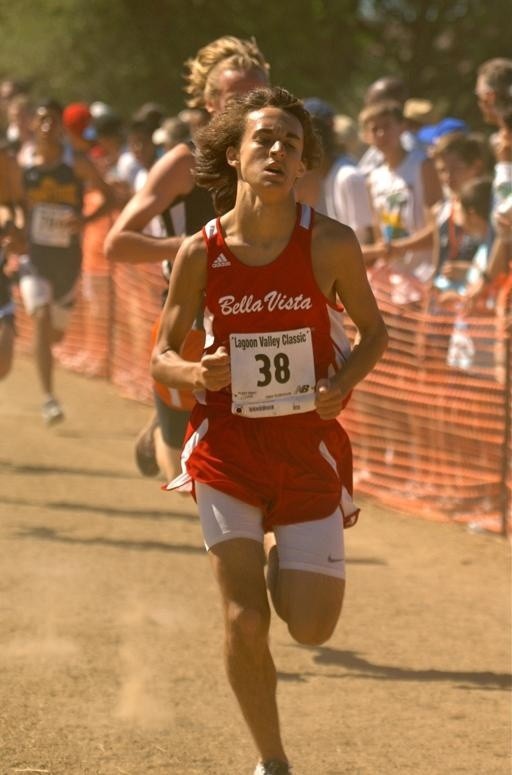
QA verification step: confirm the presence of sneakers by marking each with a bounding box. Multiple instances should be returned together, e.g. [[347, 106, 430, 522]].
[[41, 399, 63, 426], [133, 413, 160, 475]]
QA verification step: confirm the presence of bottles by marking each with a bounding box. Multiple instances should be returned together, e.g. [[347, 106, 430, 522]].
[[443, 315, 473, 370]]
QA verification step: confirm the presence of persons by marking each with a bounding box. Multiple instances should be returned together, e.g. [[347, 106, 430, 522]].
[[150, 88, 388, 775], [302, 58, 511, 509], [0, 34, 275, 485]]
